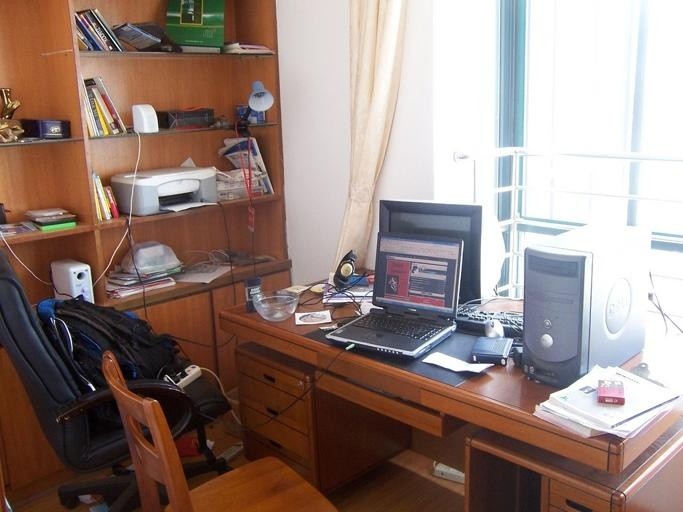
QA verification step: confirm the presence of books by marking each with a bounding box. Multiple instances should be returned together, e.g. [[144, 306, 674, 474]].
[[527, 360, 682, 442], [92, 169, 120, 222], [80, 73, 128, 138], [74, 8, 276, 55], [321, 282, 372, 305], [326, 272, 374, 297], [105, 262, 236, 299], [182, 137, 275, 201]]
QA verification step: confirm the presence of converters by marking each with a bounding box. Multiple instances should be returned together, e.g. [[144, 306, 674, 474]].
[[471, 336, 514, 367]]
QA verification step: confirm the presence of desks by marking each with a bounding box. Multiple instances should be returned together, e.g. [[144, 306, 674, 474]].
[[217, 267, 682, 512]]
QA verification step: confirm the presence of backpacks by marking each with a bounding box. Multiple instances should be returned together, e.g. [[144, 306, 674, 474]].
[[37, 297, 181, 426]]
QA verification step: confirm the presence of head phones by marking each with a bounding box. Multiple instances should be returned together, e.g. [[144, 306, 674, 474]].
[[333, 248, 357, 288]]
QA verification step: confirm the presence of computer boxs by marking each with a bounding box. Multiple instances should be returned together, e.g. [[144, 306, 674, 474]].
[[521, 225, 650, 389]]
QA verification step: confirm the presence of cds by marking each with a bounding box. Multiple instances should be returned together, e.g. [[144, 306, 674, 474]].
[[298, 313, 327, 322]]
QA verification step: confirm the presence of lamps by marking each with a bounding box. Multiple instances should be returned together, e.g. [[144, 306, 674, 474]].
[[237, 79, 276, 125]]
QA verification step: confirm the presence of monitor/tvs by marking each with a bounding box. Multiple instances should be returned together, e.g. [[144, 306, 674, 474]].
[[378, 199, 483, 305]]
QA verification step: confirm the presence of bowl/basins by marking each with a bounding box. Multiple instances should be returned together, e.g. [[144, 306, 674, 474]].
[[252, 289, 300, 323]]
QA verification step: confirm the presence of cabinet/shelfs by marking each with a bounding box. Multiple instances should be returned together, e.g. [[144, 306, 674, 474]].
[[0, 0, 293, 512]]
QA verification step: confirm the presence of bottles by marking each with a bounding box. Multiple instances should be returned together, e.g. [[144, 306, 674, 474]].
[[244, 277, 263, 314]]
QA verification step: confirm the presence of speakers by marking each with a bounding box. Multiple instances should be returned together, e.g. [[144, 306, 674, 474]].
[[51, 256, 95, 304], [131, 103, 160, 134]]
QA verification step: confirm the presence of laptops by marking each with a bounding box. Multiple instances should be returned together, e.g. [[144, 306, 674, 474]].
[[324, 231, 466, 362]]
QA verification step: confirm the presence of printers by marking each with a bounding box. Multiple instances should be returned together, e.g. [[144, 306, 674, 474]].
[[111, 166, 218, 217]]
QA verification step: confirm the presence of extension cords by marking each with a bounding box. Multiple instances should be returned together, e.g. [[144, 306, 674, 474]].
[[163, 364, 201, 388]]
